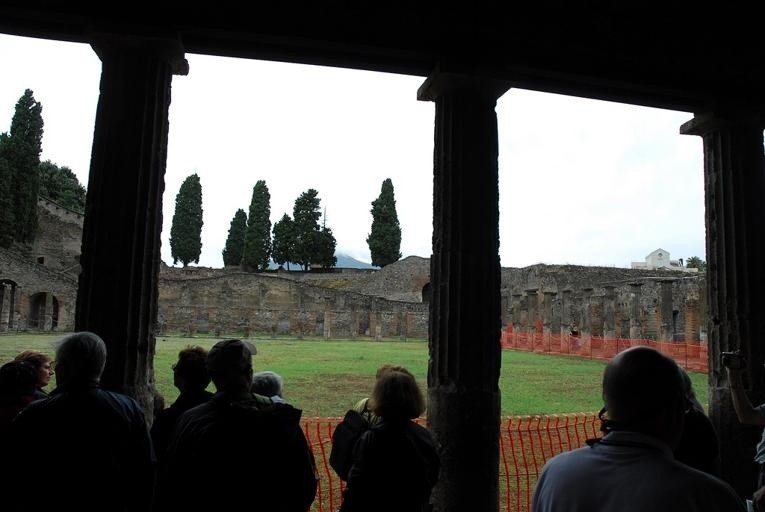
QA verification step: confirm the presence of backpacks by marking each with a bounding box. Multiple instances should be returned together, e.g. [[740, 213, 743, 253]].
[[329, 408, 374, 480]]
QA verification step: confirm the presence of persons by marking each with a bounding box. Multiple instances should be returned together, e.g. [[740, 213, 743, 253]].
[[530, 345, 765, 512], [334, 363, 418, 481], [1, 332, 153, 511], [337, 370, 441, 511], [149, 339, 319, 512]]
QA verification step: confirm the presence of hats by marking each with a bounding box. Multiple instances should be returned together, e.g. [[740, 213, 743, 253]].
[[253, 371, 282, 396], [206, 338, 257, 375]]
